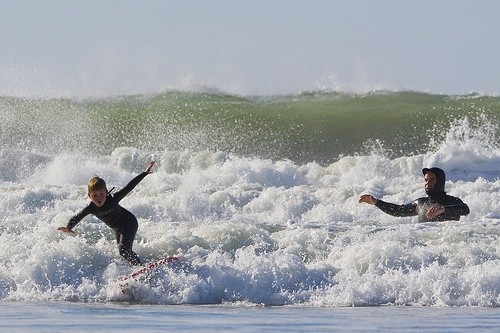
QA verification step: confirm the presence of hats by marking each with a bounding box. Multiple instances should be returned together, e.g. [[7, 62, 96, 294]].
[[420, 168, 444, 176]]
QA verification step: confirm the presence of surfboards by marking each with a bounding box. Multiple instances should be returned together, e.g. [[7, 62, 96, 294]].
[[125, 257, 176, 280]]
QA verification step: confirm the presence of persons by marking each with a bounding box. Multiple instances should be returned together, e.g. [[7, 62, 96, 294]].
[[358, 166, 470, 223], [57, 159, 156, 271]]
